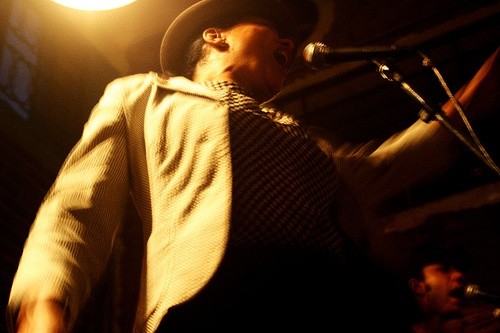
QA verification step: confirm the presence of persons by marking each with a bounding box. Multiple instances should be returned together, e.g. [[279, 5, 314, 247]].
[[389, 228, 500, 333], [1, 1, 500, 333]]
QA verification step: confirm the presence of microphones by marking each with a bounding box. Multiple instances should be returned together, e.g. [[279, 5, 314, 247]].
[[464, 284, 500, 308], [303, 41, 418, 70]]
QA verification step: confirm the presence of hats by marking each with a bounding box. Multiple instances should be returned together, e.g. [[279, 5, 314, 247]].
[[160, 1, 301, 78]]
